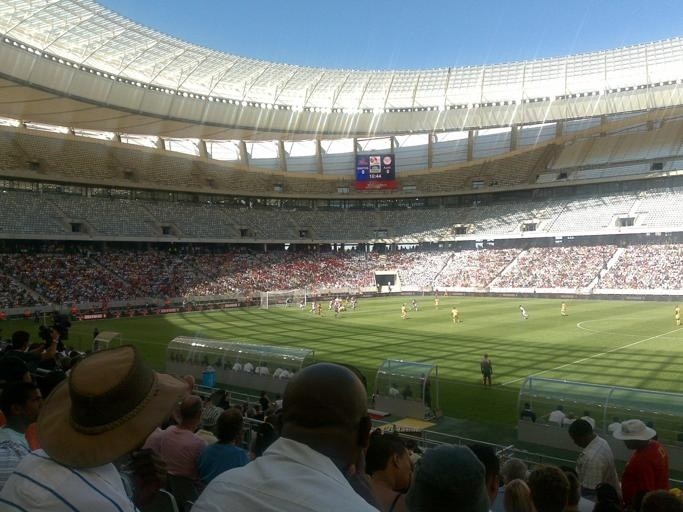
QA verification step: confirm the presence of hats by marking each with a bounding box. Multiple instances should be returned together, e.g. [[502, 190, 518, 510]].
[[404, 444, 492, 511], [38, 340, 189, 469], [200, 405, 225, 426], [613, 419, 657, 440]]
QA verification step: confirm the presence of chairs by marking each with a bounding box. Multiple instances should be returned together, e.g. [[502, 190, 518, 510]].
[[522, 415, 683, 447], [373, 393, 432, 417]]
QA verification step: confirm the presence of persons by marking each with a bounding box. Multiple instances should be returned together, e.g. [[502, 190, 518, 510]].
[[366, 235, 682, 325], [480, 352, 494, 389], [2, 238, 365, 321], [407, 402, 683, 512], [1, 325, 406, 512]]
